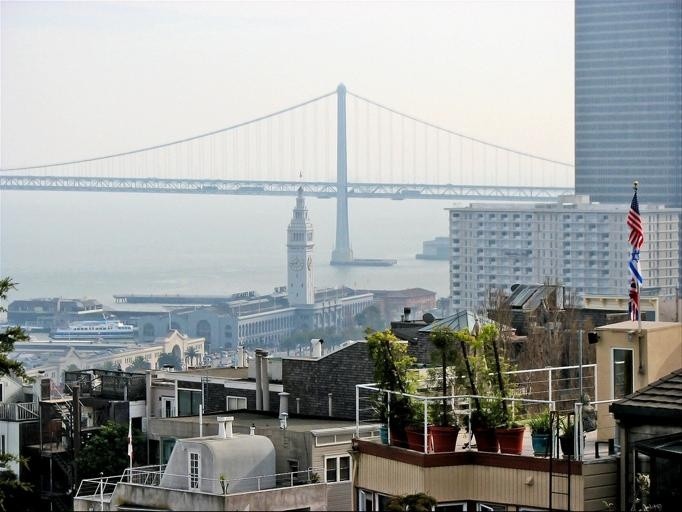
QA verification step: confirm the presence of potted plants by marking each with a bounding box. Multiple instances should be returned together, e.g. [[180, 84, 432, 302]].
[[363, 321, 525, 457], [528, 405, 586, 456]]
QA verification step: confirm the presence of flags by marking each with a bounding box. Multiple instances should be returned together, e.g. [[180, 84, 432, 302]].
[[627, 195, 644, 321]]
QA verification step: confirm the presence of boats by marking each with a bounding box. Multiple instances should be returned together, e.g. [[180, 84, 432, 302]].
[[50, 320, 135, 338]]
